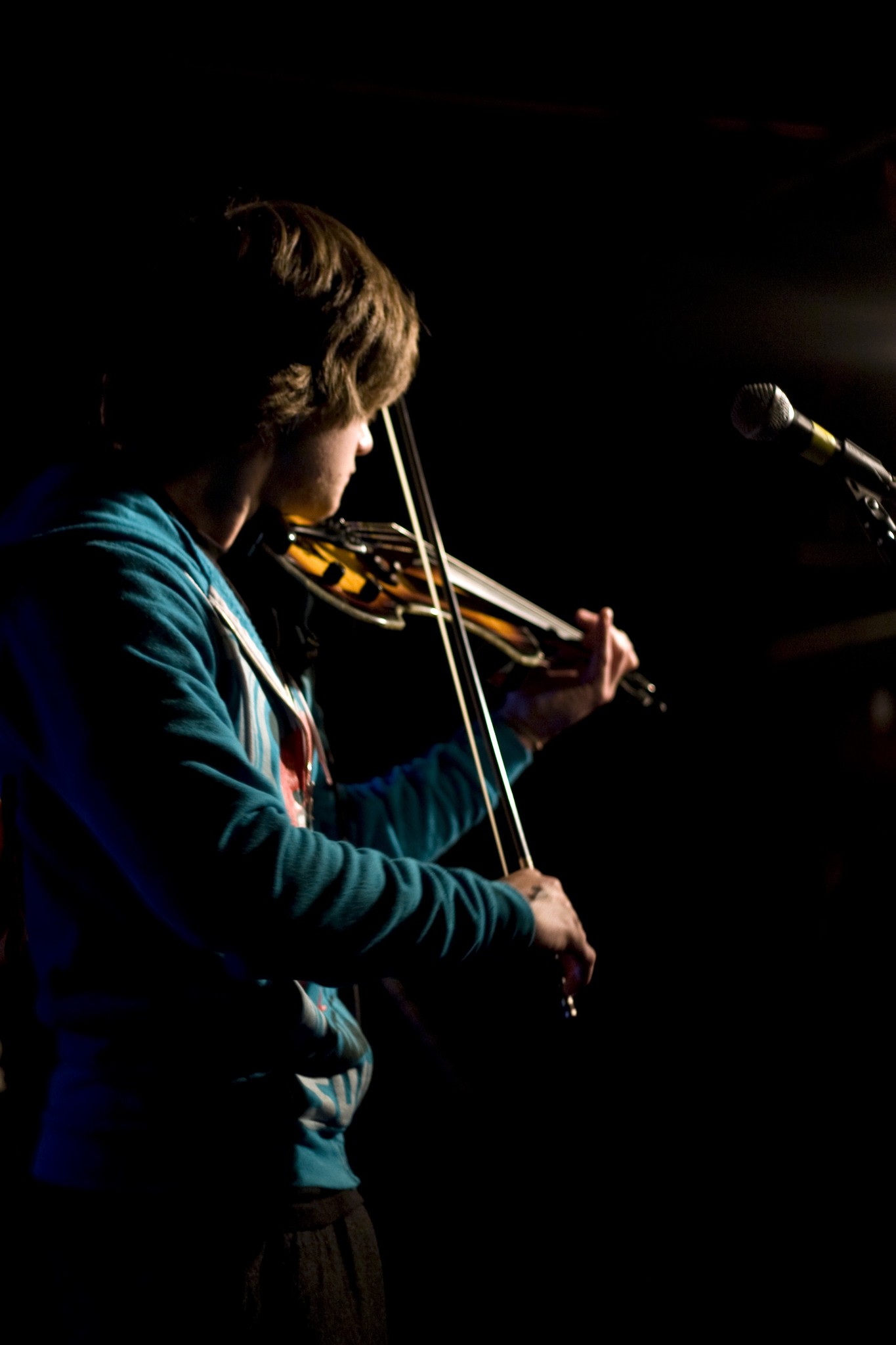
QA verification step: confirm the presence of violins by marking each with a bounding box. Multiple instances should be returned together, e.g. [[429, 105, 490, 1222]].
[[261, 517, 668, 719]]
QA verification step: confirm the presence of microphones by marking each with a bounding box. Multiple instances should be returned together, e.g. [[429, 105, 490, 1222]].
[[731, 382, 896, 504]]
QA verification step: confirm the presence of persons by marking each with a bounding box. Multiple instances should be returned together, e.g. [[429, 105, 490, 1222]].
[[0, 204, 637, 1345]]
